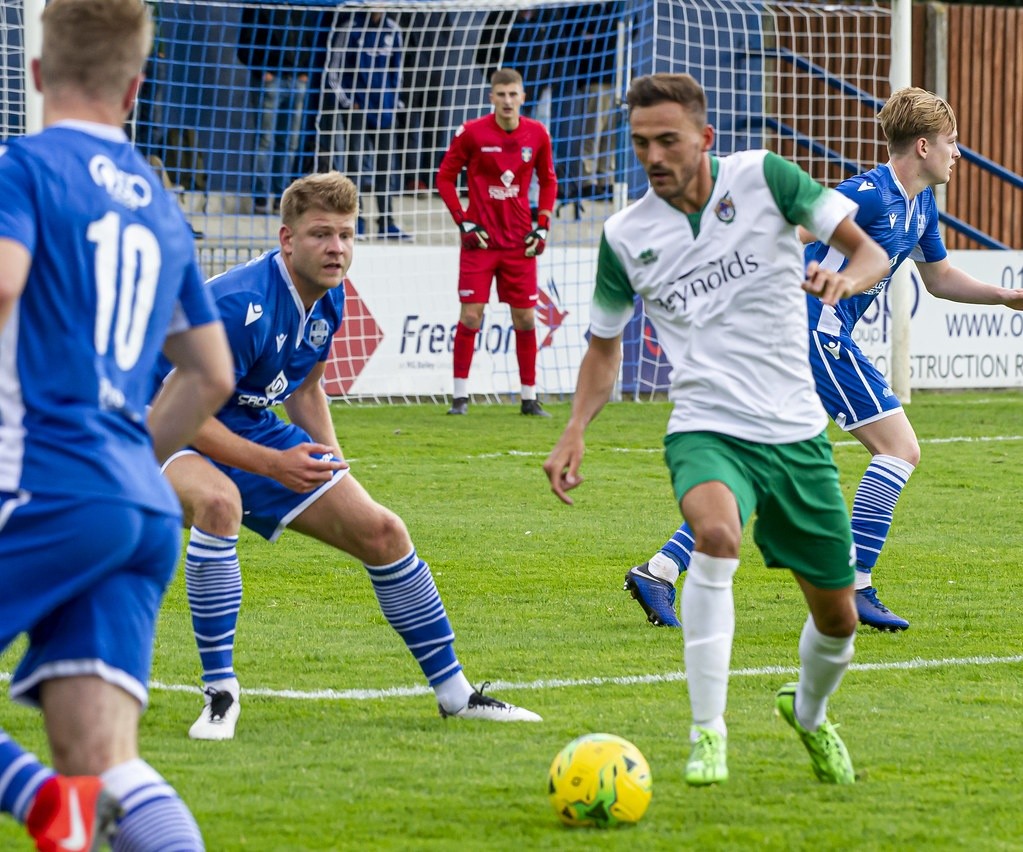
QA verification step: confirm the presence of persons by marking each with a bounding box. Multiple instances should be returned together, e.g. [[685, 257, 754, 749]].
[[435, 68, 557, 417], [626, 85, 1022, 633], [541, 72, 891, 788], [326, 11, 412, 243], [146, 171, 540, 741], [0, 0, 236, 852], [475, 1, 639, 205], [238, 1, 334, 214]]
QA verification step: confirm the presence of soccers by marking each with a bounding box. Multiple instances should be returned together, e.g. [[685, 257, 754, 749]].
[[545, 731, 654, 828]]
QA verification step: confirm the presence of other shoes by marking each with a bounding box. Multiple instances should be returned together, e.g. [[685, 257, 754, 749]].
[[255, 196, 281, 216]]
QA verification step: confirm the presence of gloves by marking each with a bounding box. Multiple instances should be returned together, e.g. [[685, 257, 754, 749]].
[[524, 215, 551, 257], [453, 213, 489, 250]]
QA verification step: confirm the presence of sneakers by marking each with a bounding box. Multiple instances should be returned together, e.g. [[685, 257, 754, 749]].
[[521, 400, 553, 419], [438, 682, 545, 722], [853, 585, 909, 632], [777, 688, 855, 786], [447, 398, 468, 414], [358, 217, 368, 242], [684, 727, 729, 785], [188, 686, 241, 741], [378, 225, 414, 243], [622, 561, 683, 629], [27, 776, 125, 852]]
